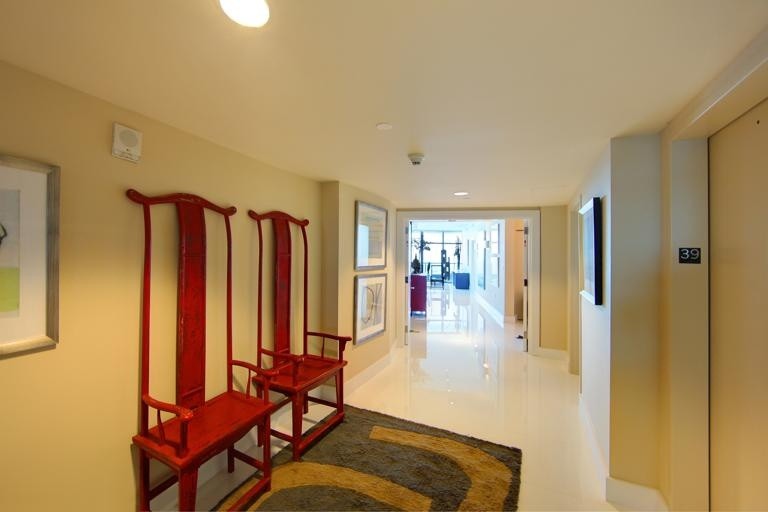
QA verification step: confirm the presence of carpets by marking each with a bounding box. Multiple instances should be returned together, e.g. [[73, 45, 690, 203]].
[[210, 403, 523, 510]]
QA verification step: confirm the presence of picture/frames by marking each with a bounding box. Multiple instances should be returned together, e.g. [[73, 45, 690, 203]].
[[577, 195, 603, 307], [352, 198, 389, 270], [354, 271, 388, 346], [1, 148, 61, 359], [475, 221, 503, 294]]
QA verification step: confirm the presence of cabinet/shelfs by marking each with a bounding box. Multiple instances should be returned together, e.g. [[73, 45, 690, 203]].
[[411, 272, 427, 315], [452, 270, 469, 291]]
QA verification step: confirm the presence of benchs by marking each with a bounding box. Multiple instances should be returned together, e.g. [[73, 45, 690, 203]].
[[430, 275, 443, 288]]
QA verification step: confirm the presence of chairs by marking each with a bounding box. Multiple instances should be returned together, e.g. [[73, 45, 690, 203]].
[[124, 186, 280, 512], [246, 207, 355, 461]]
